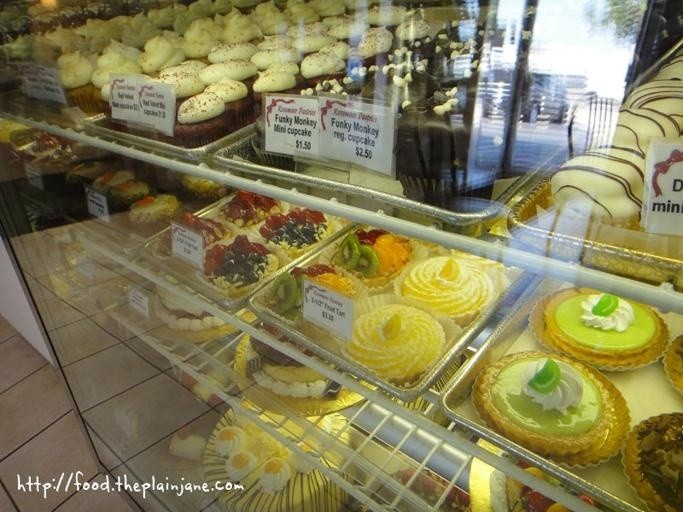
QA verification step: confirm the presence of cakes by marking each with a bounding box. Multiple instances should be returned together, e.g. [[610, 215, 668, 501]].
[[0, 0, 683, 512]]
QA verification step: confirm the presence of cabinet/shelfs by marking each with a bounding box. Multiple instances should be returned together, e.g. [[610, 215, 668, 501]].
[[0, 1, 682, 512]]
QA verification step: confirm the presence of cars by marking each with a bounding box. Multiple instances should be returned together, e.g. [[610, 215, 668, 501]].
[[452, 46, 594, 126]]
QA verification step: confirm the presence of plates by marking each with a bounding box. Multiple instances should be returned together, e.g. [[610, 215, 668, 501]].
[[469, 278, 682, 512]]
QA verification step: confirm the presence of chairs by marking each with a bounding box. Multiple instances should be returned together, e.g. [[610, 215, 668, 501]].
[[399, 67, 512, 236], [546, 95, 622, 267]]
